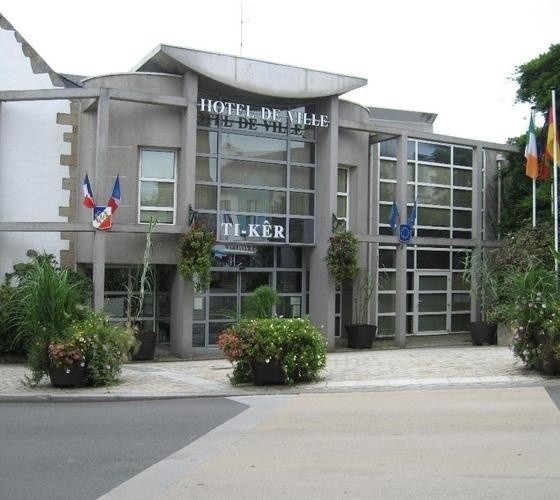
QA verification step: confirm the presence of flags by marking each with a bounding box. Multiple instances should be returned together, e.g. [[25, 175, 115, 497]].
[[543, 103, 560, 172], [80, 168, 97, 211], [522, 111, 539, 182], [106, 171, 123, 215], [404, 198, 418, 231], [387, 197, 400, 230]]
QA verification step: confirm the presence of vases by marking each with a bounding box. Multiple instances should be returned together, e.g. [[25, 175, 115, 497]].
[[46, 361, 87, 388], [247, 357, 285, 386], [542, 354, 559, 378]]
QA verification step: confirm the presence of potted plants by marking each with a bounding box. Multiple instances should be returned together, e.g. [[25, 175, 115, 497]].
[[321, 230, 361, 289], [345, 252, 390, 349], [116, 216, 158, 361], [175, 219, 218, 294], [454, 239, 498, 345]]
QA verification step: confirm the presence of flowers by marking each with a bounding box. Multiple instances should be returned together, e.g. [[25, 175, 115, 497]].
[[47, 332, 87, 368], [215, 313, 328, 386], [488, 258, 560, 370]]
